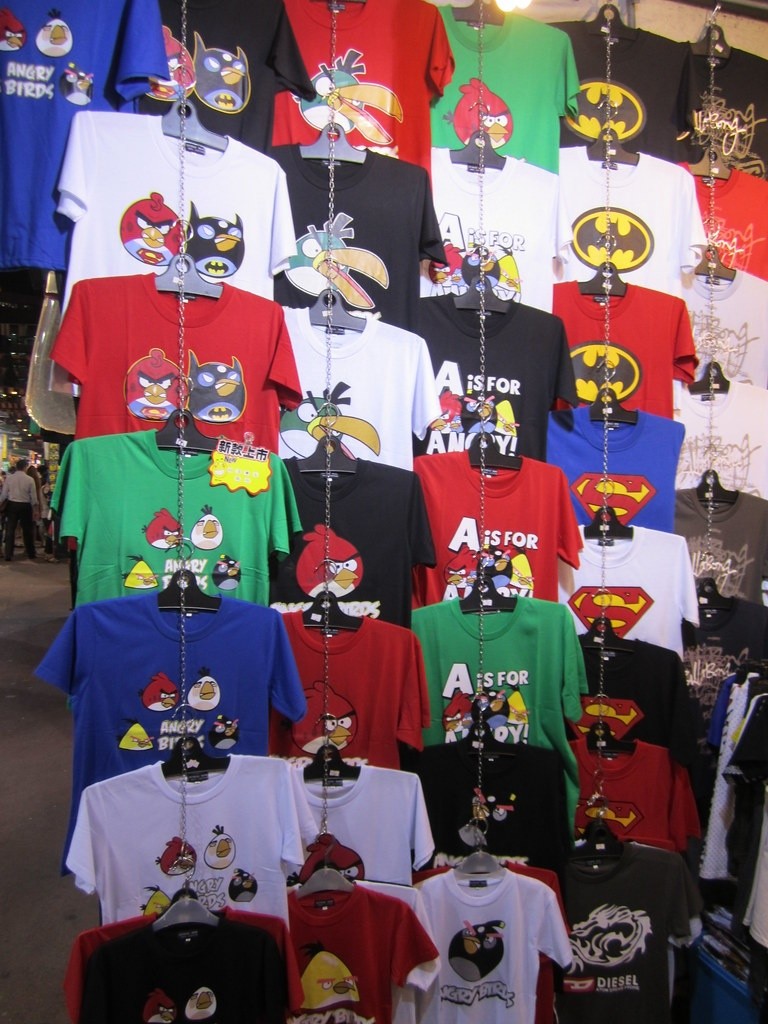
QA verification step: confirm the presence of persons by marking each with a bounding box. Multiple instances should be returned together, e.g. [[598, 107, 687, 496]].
[[0, 458, 53, 561]]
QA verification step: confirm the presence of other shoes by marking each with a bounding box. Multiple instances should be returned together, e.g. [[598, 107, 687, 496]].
[[29, 555, 36, 559], [44, 556, 60, 564], [5, 557, 11, 562]]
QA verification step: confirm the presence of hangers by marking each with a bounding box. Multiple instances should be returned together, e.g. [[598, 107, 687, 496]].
[[152, 0, 739, 935]]
